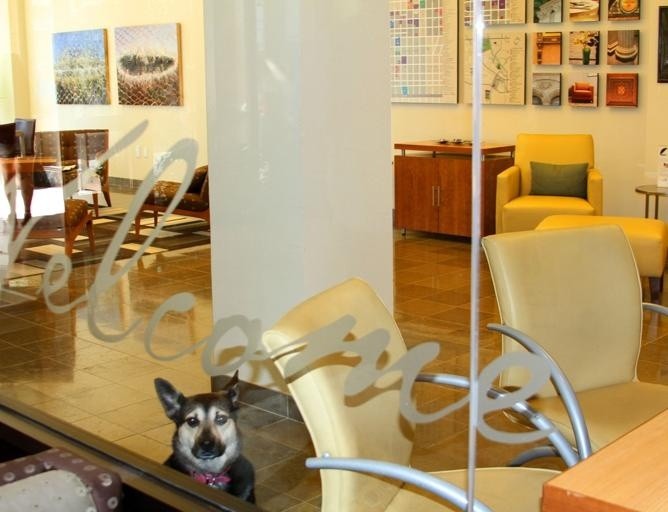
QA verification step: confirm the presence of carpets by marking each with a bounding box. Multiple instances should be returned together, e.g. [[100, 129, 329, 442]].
[[12, 210, 209, 272]]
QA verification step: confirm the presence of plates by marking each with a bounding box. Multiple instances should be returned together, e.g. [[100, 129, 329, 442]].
[[436, 140, 472, 146]]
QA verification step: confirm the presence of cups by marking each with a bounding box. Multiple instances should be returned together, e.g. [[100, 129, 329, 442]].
[[583, 49, 591, 65]]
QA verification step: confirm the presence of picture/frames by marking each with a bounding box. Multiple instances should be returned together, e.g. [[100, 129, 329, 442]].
[[604, 72, 638, 107]]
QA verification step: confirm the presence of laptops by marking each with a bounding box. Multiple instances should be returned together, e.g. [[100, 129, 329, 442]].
[[0, 118, 35, 157]]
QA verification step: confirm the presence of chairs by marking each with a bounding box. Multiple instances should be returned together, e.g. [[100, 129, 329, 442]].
[[135, 162, 209, 242], [261, 276, 579, 512], [495, 132, 603, 234], [479, 225, 668, 467], [1, 118, 112, 218]]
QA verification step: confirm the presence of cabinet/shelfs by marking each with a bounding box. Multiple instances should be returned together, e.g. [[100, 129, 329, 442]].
[[393, 140, 514, 240]]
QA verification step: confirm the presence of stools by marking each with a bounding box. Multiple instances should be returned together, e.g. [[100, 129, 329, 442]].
[[11, 199, 95, 263], [532, 213, 666, 325]]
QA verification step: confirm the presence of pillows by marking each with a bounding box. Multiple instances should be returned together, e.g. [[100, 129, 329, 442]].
[[186, 165, 207, 193], [527, 159, 588, 199]]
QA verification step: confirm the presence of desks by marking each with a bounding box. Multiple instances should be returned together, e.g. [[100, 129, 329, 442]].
[[633, 185, 666, 292], [537, 407, 668, 512]]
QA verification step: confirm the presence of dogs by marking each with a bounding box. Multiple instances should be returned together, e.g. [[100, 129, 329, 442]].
[[154, 367, 258, 505]]
